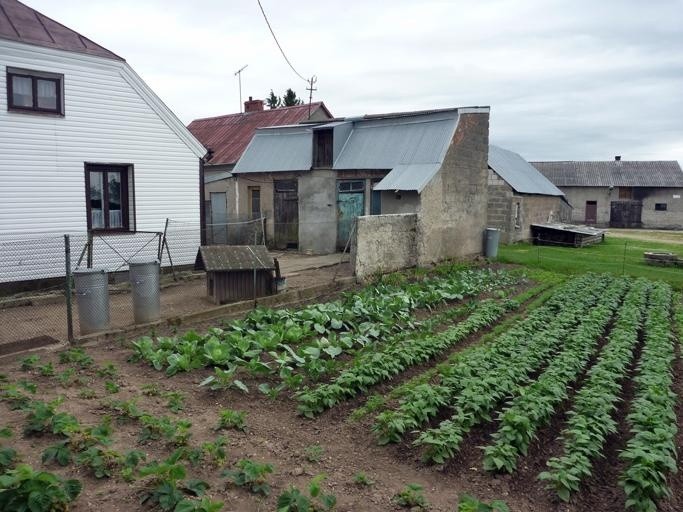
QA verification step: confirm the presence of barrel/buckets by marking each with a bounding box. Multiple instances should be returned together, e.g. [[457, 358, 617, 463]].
[[485, 227, 501, 256], [73, 268, 110, 334], [129, 260, 161, 322]]
